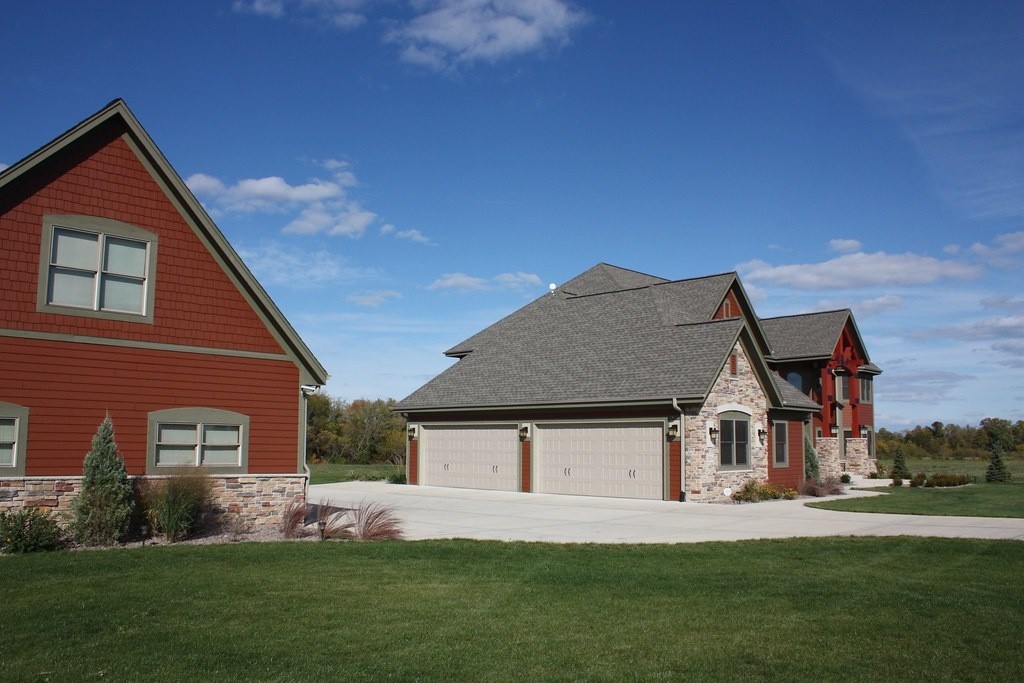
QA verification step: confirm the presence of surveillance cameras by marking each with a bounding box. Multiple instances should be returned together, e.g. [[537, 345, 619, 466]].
[[301, 385, 316, 392]]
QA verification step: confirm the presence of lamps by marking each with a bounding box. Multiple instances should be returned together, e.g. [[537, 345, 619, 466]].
[[408, 428, 415, 438], [758, 429, 767, 440], [519, 427, 528, 438], [830, 422, 839, 434], [859, 425, 868, 435], [709, 427, 719, 438], [668, 424, 678, 436]]
